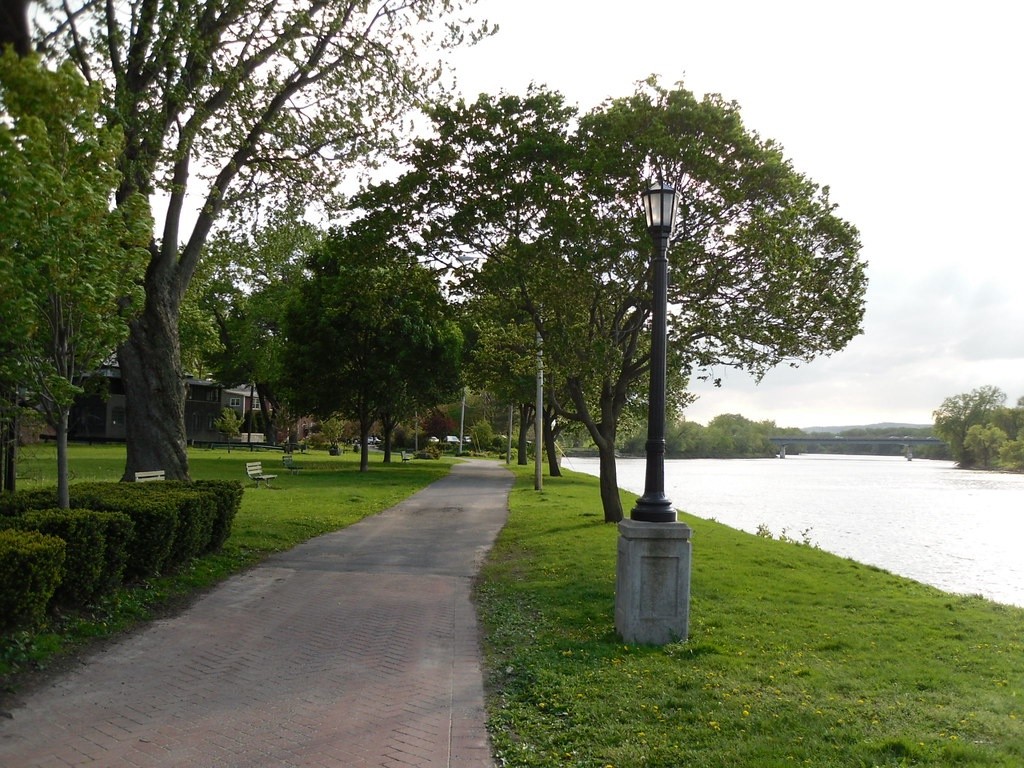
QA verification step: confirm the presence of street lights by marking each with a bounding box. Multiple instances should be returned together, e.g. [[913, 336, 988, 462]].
[[614, 176, 690, 643]]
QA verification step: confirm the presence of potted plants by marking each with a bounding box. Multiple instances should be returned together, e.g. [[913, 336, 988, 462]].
[[322, 419, 347, 455]]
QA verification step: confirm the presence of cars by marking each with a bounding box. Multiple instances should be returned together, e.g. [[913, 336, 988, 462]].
[[367, 437, 381, 444], [429, 437, 439, 442], [443, 436, 460, 443]]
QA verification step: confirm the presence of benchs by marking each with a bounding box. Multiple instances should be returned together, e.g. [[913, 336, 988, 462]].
[[246, 461, 278, 488], [283, 455, 304, 475], [402, 450, 413, 466], [134, 469, 166, 482]]
